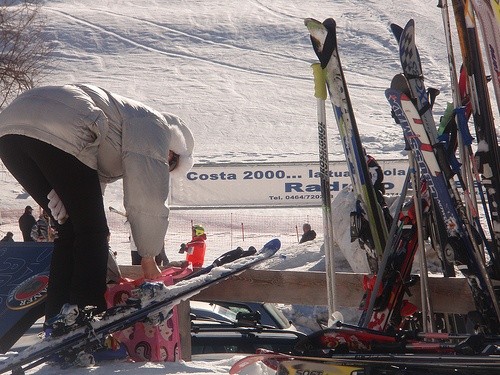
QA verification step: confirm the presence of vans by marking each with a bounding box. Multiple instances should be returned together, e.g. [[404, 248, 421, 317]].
[[212, 300, 298, 333]]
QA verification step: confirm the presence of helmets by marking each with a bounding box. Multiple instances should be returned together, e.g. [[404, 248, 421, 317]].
[[192, 224, 204, 235]]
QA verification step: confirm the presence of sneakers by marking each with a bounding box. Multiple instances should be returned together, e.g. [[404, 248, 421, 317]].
[[43, 323, 126, 362]]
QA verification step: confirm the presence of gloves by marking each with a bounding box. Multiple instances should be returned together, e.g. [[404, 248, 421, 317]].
[[47, 189, 68, 225]]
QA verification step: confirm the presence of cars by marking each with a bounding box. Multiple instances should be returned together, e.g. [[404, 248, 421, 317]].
[[189, 301, 240, 353]]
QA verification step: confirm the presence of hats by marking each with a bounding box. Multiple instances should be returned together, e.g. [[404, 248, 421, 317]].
[[25, 206, 33, 210]]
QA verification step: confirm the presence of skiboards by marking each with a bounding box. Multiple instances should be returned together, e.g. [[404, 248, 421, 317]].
[[0, 238, 281, 375], [228, 0, 500, 375]]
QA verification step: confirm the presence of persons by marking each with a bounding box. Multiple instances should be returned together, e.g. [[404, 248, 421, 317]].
[[19, 205, 37, 243], [180, 224, 209, 270], [1, 83, 197, 375], [296, 223, 319, 252]]
